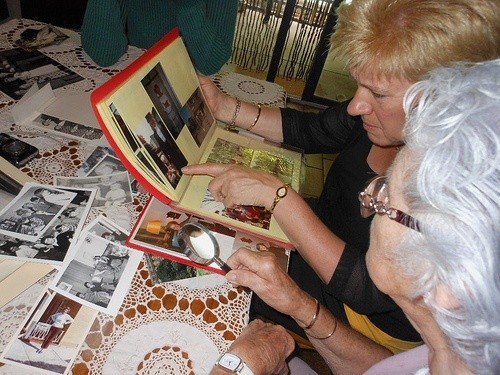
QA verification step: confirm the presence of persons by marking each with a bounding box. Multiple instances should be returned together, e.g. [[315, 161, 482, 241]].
[[207, 58, 500, 375], [103, 231, 128, 241], [182, 0, 500, 355], [0, 189, 88, 262], [76, 255, 129, 308], [35, 306, 73, 353], [167, 221, 180, 230], [80, 0, 238, 75], [154, 84, 183, 134], [94, 177, 126, 206], [41, 114, 102, 136], [0, 50, 84, 96], [137, 112, 188, 189], [256, 243, 288, 267]]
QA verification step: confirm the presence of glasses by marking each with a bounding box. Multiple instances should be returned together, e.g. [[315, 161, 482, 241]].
[[357, 174, 420, 232]]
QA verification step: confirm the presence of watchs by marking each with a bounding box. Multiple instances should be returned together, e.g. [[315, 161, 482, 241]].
[[269, 183, 289, 212], [215, 352, 254, 375]]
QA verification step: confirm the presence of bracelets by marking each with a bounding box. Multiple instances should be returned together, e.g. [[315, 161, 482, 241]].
[[247, 104, 261, 130], [305, 315, 337, 340], [226, 97, 241, 125], [302, 298, 320, 329]]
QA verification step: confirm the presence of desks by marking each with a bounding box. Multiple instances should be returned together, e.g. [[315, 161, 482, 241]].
[[0, 16, 287, 375]]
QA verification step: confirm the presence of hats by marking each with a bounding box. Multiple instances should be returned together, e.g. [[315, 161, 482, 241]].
[[145, 112, 153, 123]]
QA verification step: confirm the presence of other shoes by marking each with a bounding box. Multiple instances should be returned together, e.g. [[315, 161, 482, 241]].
[[36, 349, 39, 352], [39, 350, 42, 353]]
